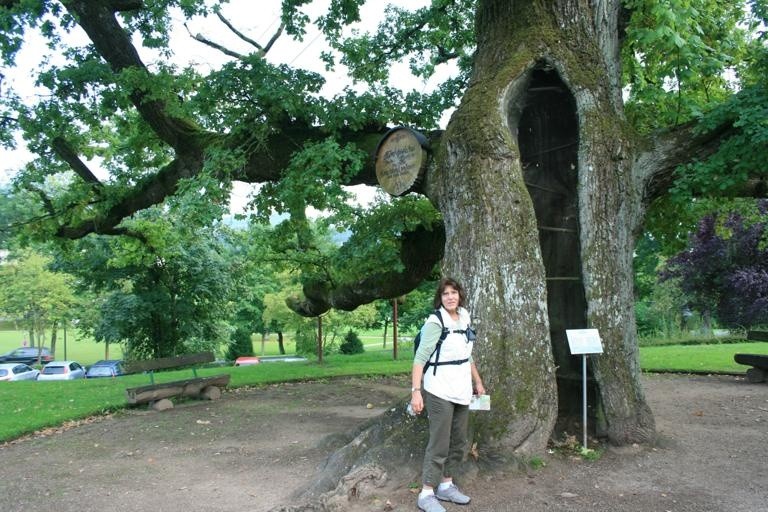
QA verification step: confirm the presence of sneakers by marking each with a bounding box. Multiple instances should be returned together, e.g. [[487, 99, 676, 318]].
[[436, 485, 471, 505], [417, 494, 446, 512]]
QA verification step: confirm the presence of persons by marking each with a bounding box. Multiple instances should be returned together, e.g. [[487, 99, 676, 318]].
[[410, 278, 491, 512]]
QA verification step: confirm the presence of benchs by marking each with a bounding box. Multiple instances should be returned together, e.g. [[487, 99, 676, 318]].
[[734, 330, 767, 382], [123, 350, 231, 411]]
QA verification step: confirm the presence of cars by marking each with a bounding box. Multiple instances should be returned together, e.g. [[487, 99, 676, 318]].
[[233, 356, 259, 367], [0, 347, 124, 381]]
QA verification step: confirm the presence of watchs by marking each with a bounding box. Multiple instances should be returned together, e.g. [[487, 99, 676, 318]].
[[411, 387, 422, 392]]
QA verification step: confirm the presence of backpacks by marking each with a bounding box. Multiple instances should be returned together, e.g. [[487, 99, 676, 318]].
[[412, 310, 444, 374]]
